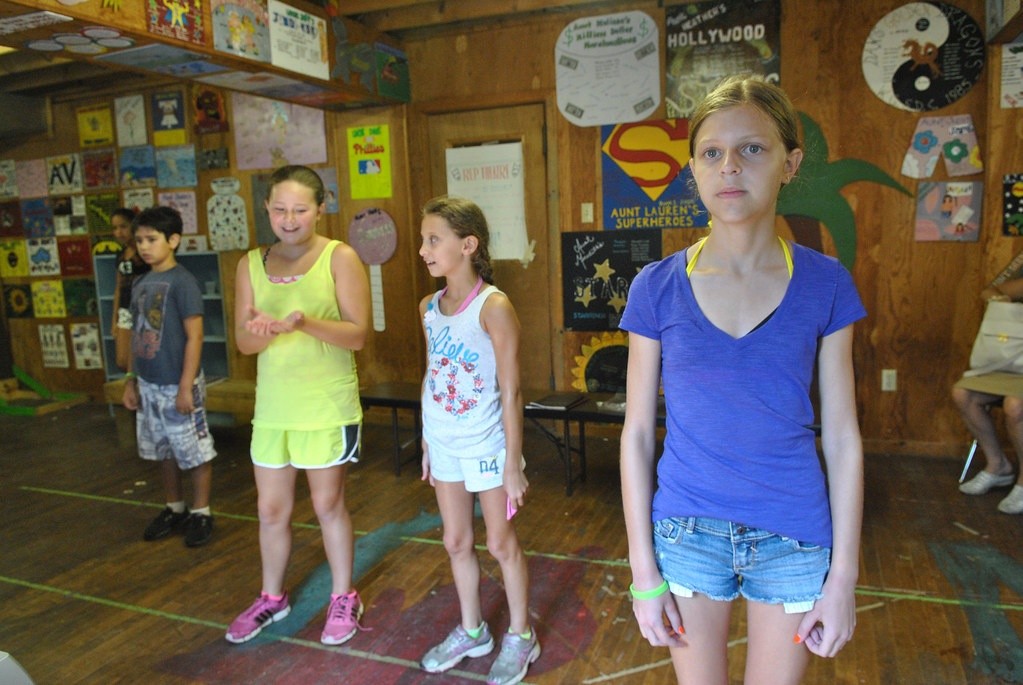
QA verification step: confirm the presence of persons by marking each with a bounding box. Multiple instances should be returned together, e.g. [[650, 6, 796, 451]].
[[419, 195, 542, 685], [623, 73, 862, 685], [224, 168, 373, 647], [953, 235, 1023, 515], [109, 208, 151, 370], [123, 205, 214, 548]]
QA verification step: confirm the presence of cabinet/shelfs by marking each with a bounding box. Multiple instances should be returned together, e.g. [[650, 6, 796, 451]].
[[92, 252, 247, 419]]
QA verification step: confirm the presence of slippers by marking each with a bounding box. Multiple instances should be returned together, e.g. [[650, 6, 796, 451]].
[[959, 470, 1016, 495], [999, 485, 1023, 514]]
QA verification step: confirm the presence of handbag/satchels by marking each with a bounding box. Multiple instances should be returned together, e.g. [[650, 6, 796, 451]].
[[963, 298, 1022, 380]]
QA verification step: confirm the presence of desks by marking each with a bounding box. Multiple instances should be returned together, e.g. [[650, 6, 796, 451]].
[[357, 382, 822, 498]]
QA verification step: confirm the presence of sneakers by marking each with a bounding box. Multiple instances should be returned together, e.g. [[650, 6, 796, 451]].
[[225, 592, 290, 643], [320, 587, 373, 646], [142, 507, 188, 540], [421, 620, 494, 673], [185, 512, 213, 547], [486, 623, 541, 685]]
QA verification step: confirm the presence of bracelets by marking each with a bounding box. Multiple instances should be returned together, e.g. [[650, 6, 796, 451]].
[[629, 580, 669, 600]]
